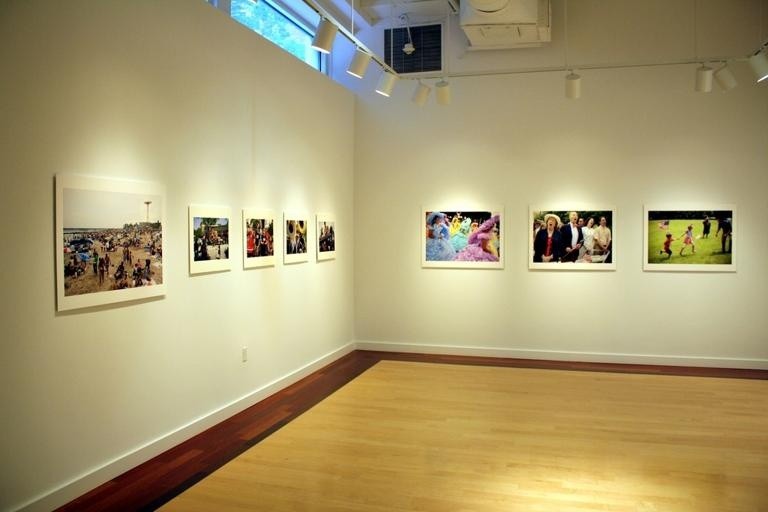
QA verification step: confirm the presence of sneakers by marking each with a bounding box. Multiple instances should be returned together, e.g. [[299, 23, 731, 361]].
[[660, 249, 662, 254]]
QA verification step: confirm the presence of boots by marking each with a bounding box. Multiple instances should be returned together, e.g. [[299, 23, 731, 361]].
[[692, 245, 696, 253], [680, 248, 685, 256]]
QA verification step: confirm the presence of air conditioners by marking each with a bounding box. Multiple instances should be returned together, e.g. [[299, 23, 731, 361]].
[[459, 0, 551, 47]]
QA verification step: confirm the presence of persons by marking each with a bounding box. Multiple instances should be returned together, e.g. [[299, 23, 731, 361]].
[[679, 226, 697, 256], [715, 214, 732, 254], [318, 221, 335, 252], [534, 211, 611, 262], [193, 229, 229, 261], [660, 233, 680, 259], [702, 215, 711, 239], [426, 212, 500, 262], [248, 223, 274, 257], [658, 220, 669, 231], [287, 232, 306, 254], [63, 226, 162, 291]]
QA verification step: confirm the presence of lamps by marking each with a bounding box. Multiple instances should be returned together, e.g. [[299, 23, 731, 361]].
[[312, 15, 338, 54], [713, 61, 739, 94], [749, 53, 768, 83], [435, 76, 450, 104], [375, 65, 396, 97], [346, 44, 371, 79], [411, 78, 430, 108], [399, 14, 415, 55], [695, 62, 713, 95], [565, 69, 581, 99]]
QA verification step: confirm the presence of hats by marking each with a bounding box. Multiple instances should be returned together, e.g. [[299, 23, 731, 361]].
[[544, 214, 560, 228]]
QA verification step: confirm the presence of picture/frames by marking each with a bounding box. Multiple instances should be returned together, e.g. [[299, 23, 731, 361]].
[[188, 204, 232, 275], [242, 209, 275, 269], [643, 203, 738, 272], [55, 174, 167, 314], [283, 210, 309, 264], [527, 205, 617, 272], [421, 205, 504, 269], [316, 213, 337, 261]]
